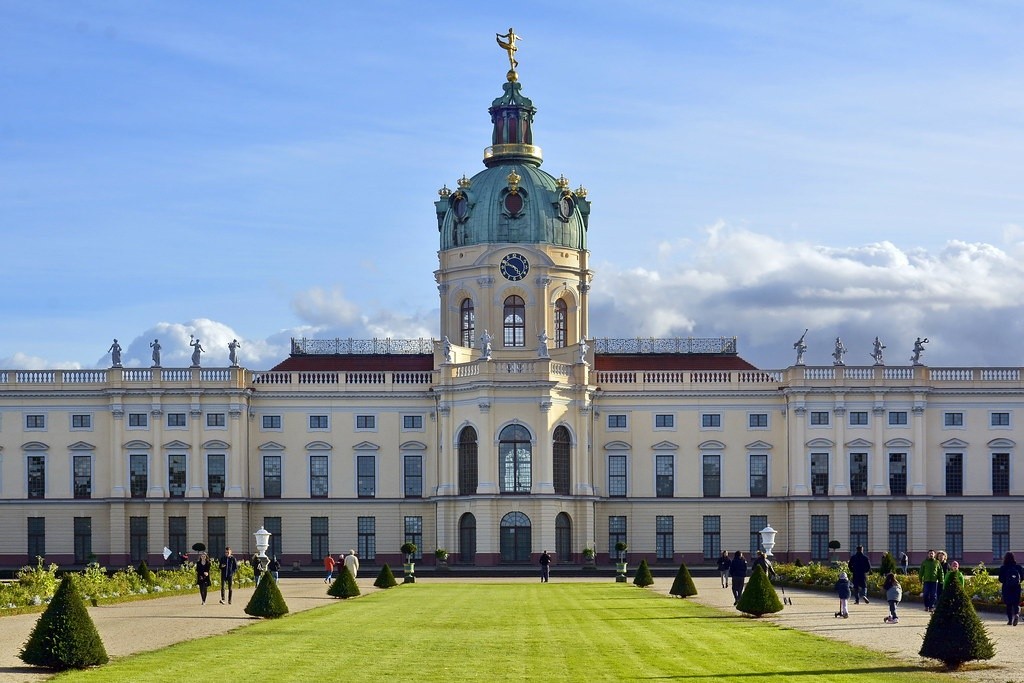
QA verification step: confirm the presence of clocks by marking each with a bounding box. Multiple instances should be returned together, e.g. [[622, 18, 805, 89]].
[[498, 252, 531, 283]]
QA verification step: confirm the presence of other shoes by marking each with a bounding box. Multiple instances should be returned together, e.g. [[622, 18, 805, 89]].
[[324, 580, 327, 584], [924, 607, 928, 611], [220, 600, 224, 604], [228, 600, 231, 604], [734, 601, 738, 605], [843, 613, 848, 618], [202, 602, 205, 605], [722, 584, 725, 588], [893, 618, 898, 623], [854, 602, 859, 604], [863, 596, 870, 603], [726, 583, 728, 588], [1013, 614, 1019, 625], [929, 607, 933, 612], [1007, 622, 1012, 625]]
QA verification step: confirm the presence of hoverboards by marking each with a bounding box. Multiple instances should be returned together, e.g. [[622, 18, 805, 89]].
[[884, 610, 898, 624], [835, 598, 844, 618]]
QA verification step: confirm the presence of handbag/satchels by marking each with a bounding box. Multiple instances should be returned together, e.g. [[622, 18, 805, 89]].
[[257, 559, 264, 571]]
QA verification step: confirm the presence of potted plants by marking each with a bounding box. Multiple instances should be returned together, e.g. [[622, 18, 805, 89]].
[[582, 548, 595, 561], [435, 548, 449, 562], [828, 540, 841, 569], [614, 541, 629, 583], [400, 542, 418, 583]]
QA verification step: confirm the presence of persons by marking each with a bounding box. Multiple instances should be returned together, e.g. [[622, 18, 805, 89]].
[[536, 329, 554, 357], [251, 553, 280, 588], [539, 550, 551, 582], [835, 544, 871, 618], [793, 337, 808, 364], [196, 552, 212, 605], [718, 550, 778, 606], [998, 551, 1024, 626], [870, 335, 883, 364], [443, 335, 452, 361], [481, 329, 494, 358], [898, 551, 909, 575], [577, 335, 590, 363], [918, 549, 965, 612], [832, 337, 845, 364], [190, 339, 205, 365], [228, 339, 241, 366], [108, 339, 122, 366], [177, 550, 189, 565], [910, 337, 924, 364], [497, 27, 523, 70], [219, 547, 237, 604], [324, 549, 359, 585], [150, 339, 162, 366], [881, 573, 902, 623]]
